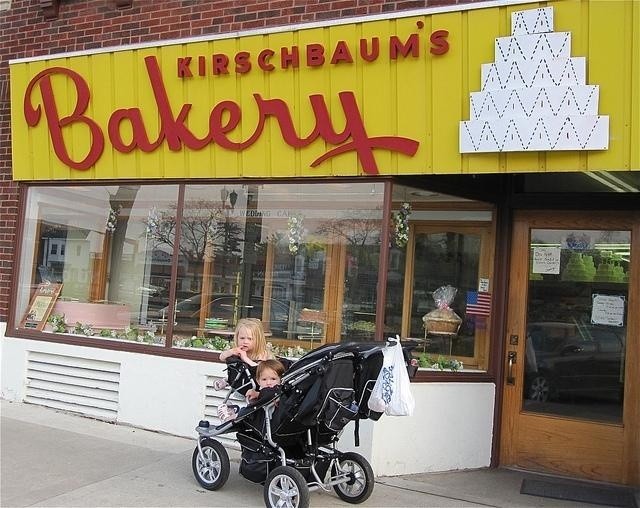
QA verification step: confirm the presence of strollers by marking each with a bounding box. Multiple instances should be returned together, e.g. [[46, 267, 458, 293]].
[[191, 339, 420, 508]]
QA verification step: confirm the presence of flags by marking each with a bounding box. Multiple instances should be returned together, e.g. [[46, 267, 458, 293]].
[[466, 291, 491, 316]]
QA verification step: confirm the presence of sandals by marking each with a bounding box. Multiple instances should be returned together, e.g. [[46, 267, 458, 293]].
[[217, 401, 236, 423], [213, 378, 230, 391]]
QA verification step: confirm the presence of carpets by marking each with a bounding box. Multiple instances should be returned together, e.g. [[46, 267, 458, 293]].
[[518, 477, 638, 507]]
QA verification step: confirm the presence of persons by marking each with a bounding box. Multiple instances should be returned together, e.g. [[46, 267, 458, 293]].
[[213, 318, 276, 391], [217, 360, 285, 424]]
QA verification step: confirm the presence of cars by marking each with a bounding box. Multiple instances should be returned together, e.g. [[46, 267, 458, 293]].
[[119, 293, 323, 341], [458, 320, 625, 402]]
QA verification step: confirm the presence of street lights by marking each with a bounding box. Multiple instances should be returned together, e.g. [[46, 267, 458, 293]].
[[221, 188, 237, 292]]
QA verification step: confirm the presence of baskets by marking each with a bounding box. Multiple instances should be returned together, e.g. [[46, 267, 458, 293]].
[[425, 317, 460, 332]]
